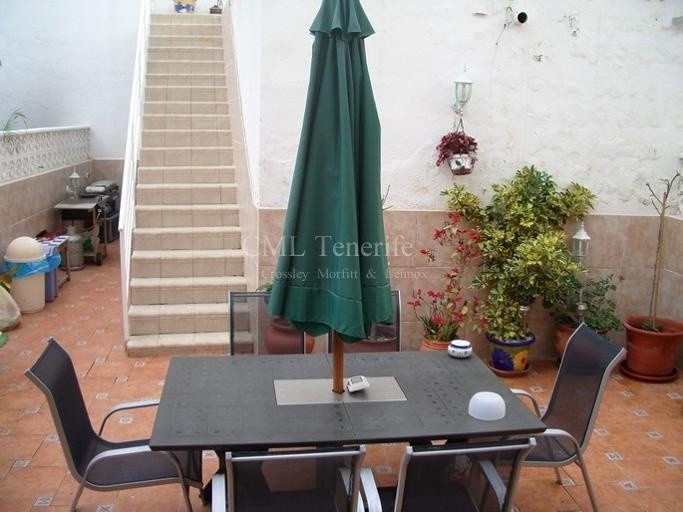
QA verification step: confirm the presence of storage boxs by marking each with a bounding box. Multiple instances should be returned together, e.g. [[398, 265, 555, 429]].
[[99, 213, 119, 241]]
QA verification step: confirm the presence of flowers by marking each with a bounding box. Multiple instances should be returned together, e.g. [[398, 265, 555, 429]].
[[408, 210, 494, 339], [435, 133, 477, 164]]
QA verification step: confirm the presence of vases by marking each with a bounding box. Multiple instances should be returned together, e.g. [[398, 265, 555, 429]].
[[448, 152, 472, 177]]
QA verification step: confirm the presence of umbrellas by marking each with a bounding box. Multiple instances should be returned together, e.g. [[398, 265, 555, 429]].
[[264, 0, 398, 393]]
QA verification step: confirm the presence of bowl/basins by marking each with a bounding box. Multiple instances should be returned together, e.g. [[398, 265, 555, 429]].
[[469, 391, 506, 424]]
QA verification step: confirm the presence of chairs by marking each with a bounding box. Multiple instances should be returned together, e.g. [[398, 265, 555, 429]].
[[327, 289, 403, 354], [379, 436, 537, 512], [467, 320, 626, 512], [22, 336, 208, 512], [210, 443, 385, 512], [227, 291, 307, 356]]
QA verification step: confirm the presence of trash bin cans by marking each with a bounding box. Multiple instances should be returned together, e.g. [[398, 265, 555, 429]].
[[3, 236, 59, 314]]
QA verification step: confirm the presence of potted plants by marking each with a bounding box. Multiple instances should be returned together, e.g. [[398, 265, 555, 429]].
[[434, 165, 596, 380], [540, 272, 624, 370], [623, 167, 683, 381], [255, 280, 316, 354]]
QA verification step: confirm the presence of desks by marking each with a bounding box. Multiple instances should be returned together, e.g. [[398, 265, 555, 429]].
[[148, 347, 550, 497]]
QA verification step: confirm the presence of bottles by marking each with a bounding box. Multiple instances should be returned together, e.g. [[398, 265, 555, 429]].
[[447, 339, 474, 358]]
